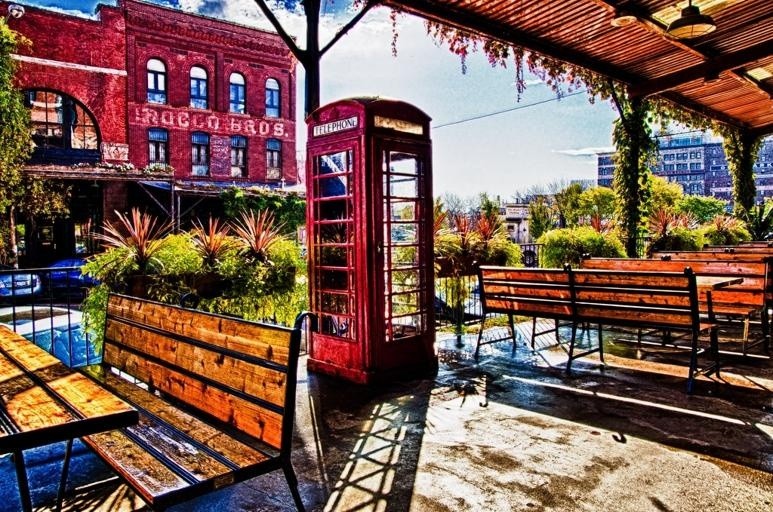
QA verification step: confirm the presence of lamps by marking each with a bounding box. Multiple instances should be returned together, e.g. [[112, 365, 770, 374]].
[[665, 0, 717, 40]]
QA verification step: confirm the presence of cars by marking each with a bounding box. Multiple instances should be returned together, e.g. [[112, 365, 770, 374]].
[[433, 281, 502, 326], [519, 242, 540, 267], [0, 262, 41, 301], [40, 258, 100, 301], [0, 307, 162, 402], [389, 228, 409, 242]]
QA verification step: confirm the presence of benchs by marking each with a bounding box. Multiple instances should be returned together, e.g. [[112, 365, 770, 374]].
[[68, 289, 317, 512]]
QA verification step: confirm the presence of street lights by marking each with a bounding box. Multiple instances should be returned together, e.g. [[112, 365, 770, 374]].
[[4, 5, 24, 24], [521, 228, 527, 243]]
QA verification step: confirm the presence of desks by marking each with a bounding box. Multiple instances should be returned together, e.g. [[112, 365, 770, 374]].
[[0, 325, 136, 511]]
